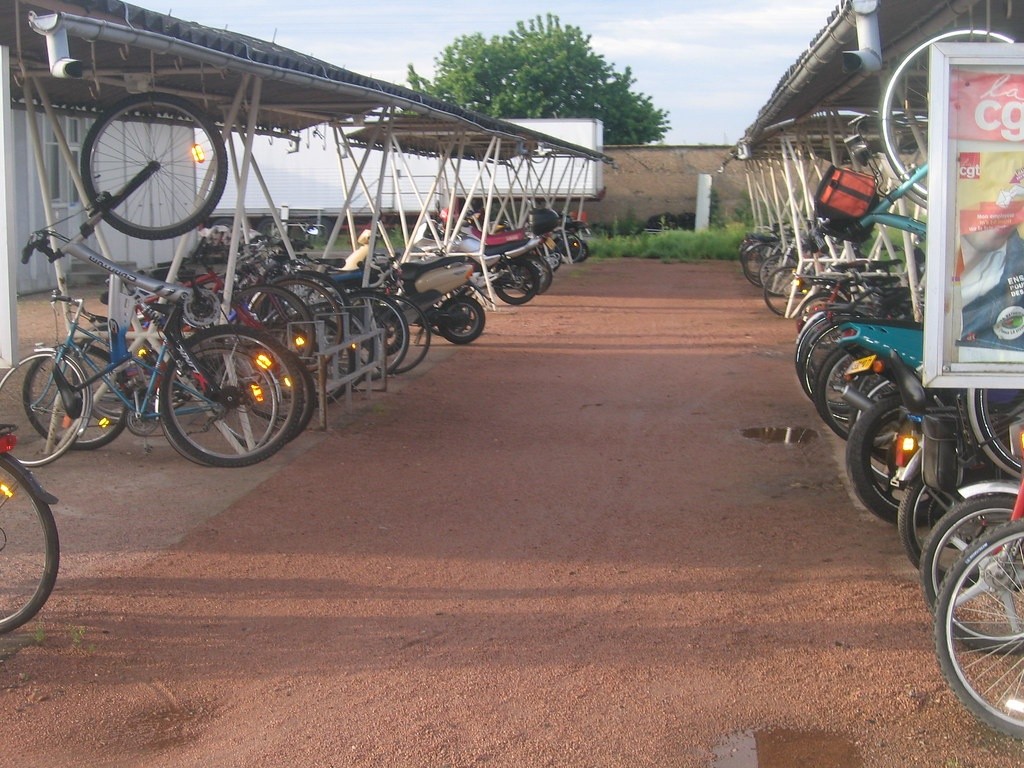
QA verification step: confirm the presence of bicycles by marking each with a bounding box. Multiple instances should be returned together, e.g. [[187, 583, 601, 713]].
[[0, 93, 589, 467], [0, 420, 61, 635], [735, 25, 1024, 740]]
